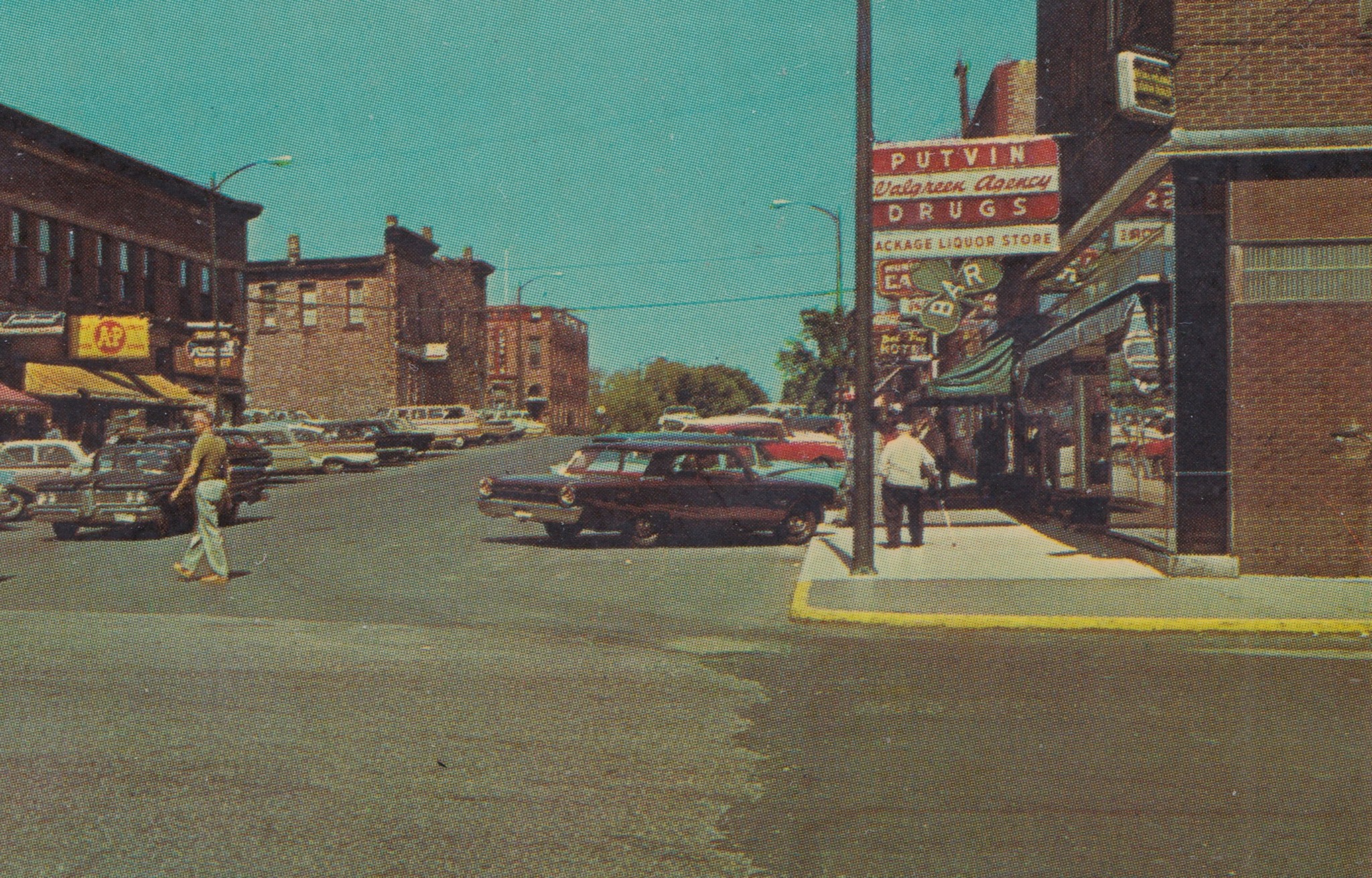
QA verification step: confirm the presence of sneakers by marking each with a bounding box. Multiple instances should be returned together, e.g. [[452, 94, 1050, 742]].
[[173, 561, 193, 583], [201, 571, 231, 584]]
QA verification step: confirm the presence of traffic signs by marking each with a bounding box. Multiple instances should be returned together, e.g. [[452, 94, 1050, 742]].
[[873, 134, 1060, 258]]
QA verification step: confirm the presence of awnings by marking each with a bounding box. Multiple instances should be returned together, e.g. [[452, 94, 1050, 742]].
[[905, 329, 1015, 407]]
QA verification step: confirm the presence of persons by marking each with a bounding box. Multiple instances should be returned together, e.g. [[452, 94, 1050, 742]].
[[878, 422, 942, 549], [167, 412, 230, 584], [7, 410, 62, 440], [925, 415, 956, 499], [970, 416, 1001, 509]]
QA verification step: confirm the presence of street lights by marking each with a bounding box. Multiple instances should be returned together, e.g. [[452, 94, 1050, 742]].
[[638, 353, 663, 378], [768, 198, 844, 327], [516, 268, 563, 412], [207, 153, 296, 428]]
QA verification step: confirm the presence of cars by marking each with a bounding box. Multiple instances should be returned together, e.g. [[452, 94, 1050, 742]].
[[34, 442, 270, 542], [0, 440, 95, 495], [115, 403, 548, 480], [999, 373, 1176, 489], [549, 401, 850, 512], [479, 440, 838, 549]]
